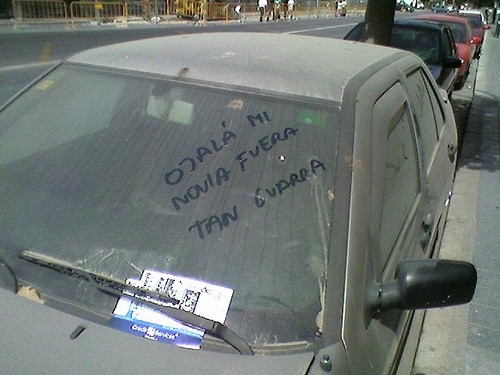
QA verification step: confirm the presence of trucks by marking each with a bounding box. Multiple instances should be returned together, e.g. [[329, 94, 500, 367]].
[[396, 0, 424, 9]]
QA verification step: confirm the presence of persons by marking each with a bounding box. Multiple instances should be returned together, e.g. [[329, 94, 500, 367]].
[[397, 0, 491, 22], [337, 1, 348, 16], [287, 0, 295, 18], [257, 0, 268, 22]]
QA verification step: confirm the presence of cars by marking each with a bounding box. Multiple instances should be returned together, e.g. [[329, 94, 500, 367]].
[[431, 4, 449, 13], [1, 31, 477, 372], [396, 3, 415, 12], [413, 14, 481, 90], [458, 9, 485, 27], [344, 16, 464, 97], [446, 10, 490, 58]]
